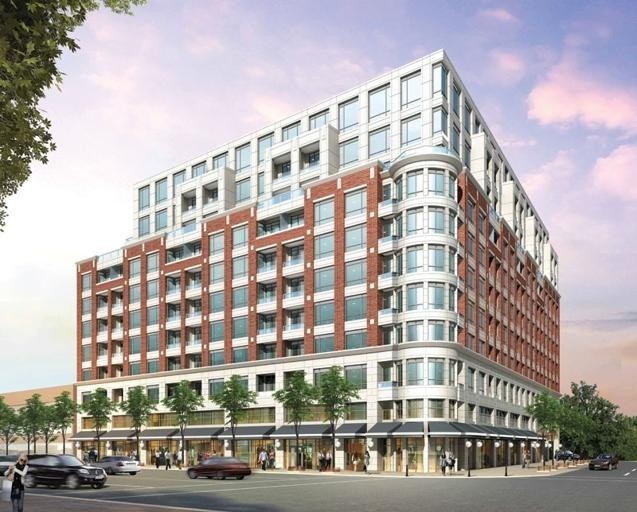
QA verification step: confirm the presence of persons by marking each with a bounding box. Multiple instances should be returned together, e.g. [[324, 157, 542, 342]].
[[3, 452, 30, 511], [154, 449, 162, 469], [521, 450, 527, 468], [439, 451, 448, 476], [211, 450, 217, 458], [258, 449, 268, 471], [446, 450, 454, 475], [318, 450, 326, 472], [351, 451, 360, 473], [175, 448, 183, 470], [362, 451, 371, 473], [325, 450, 333, 471], [164, 449, 171, 471], [268, 449, 275, 469], [84, 445, 99, 463], [525, 450, 532, 468]]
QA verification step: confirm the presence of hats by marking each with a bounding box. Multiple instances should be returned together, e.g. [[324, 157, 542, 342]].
[[589, 452, 619, 471], [0, 453, 139, 490], [186, 456, 253, 480], [555, 450, 580, 461]]
